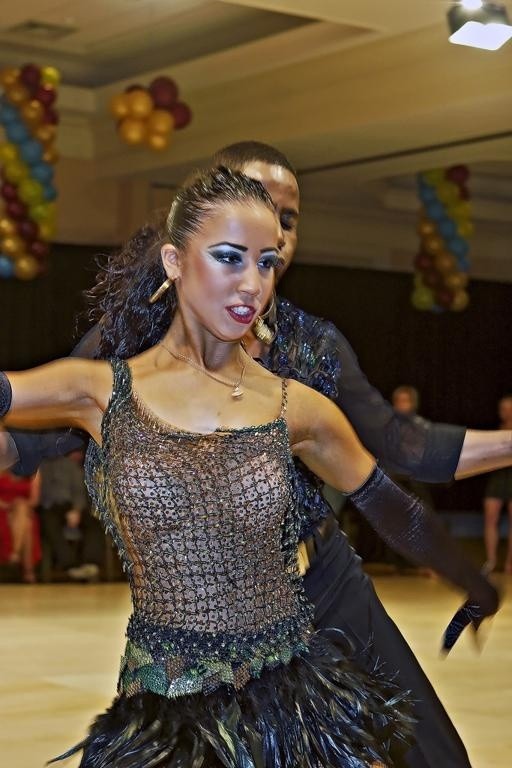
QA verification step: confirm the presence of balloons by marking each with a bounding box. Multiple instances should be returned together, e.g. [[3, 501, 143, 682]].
[[405, 163, 473, 314], [108, 75, 194, 151], [2, 62, 68, 283]]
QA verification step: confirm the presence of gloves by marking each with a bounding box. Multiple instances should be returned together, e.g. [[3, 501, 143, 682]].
[[342, 458, 501, 663]]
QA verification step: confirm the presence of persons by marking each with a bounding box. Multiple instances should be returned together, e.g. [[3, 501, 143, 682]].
[[1, 428, 128, 585], [321, 385, 512, 577]]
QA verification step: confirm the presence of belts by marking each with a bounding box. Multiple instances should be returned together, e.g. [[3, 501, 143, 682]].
[[286, 513, 336, 579]]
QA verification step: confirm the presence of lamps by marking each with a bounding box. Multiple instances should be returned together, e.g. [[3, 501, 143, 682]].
[[446, 0, 512, 53]]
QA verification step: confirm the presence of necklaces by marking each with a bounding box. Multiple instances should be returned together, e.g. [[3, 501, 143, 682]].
[[155, 336, 252, 401]]
